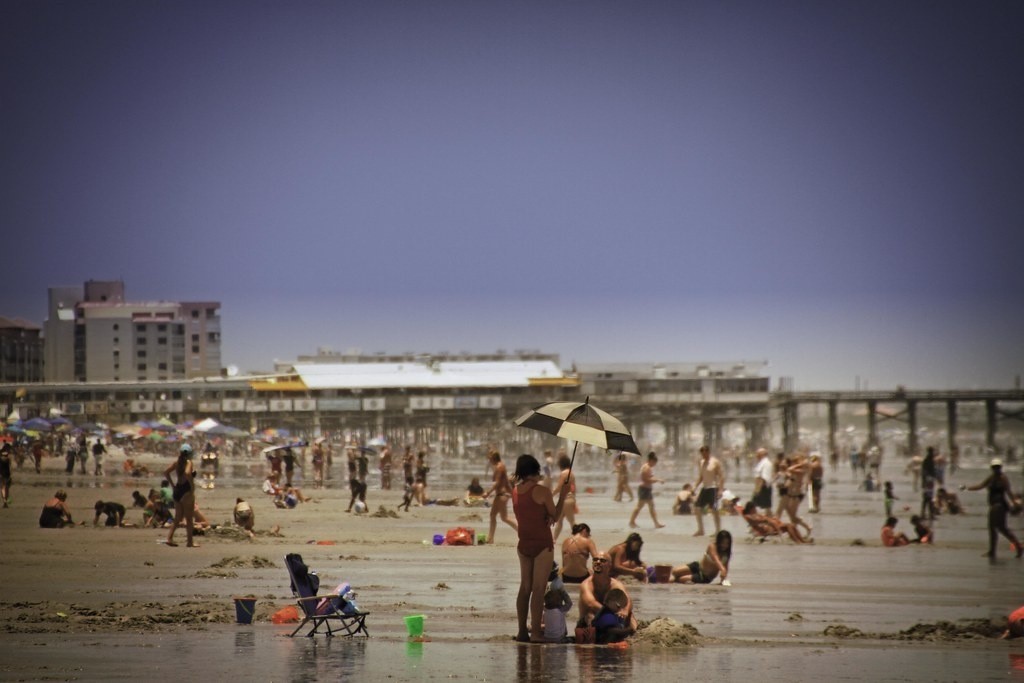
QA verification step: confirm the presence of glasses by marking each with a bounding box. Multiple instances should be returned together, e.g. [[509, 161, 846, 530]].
[[593, 558, 606, 563], [616, 602, 621, 609]]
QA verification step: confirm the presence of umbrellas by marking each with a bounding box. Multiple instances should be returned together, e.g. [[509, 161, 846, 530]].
[[0, 410, 307, 456], [512, 396, 642, 483], [368, 437, 387, 447]]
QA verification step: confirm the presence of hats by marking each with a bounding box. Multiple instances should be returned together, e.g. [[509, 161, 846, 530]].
[[990, 459, 1002, 466], [180, 444, 193, 455]]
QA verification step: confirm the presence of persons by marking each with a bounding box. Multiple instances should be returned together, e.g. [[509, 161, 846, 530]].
[[593, 588, 636, 644], [669, 529, 731, 585], [527, 561, 573, 633], [0, 430, 309, 532], [163, 444, 201, 547], [576, 552, 638, 633], [562, 523, 597, 583], [439, 429, 1023, 559], [512, 454, 570, 643], [608, 532, 647, 581], [311, 430, 434, 514], [1000, 606, 1024, 640], [542, 591, 568, 642]]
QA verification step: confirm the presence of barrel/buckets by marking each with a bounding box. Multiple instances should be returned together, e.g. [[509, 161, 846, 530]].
[[478, 534, 486, 544], [433, 534, 443, 545], [233, 597, 257, 624], [654, 564, 672, 585], [403, 615, 424, 637]]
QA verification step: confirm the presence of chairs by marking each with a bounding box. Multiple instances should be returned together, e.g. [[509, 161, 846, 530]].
[[283, 554, 371, 639], [733, 505, 788, 544]]
